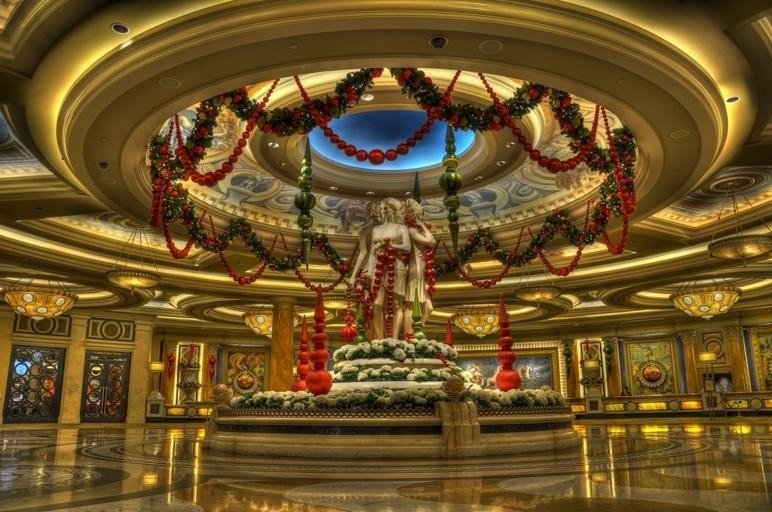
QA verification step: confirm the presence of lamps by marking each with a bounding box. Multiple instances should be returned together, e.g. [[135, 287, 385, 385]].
[[512, 257, 563, 308], [699, 350, 717, 385], [669, 278, 741, 322], [106, 226, 161, 296], [449, 304, 501, 338], [2, 276, 78, 321], [707, 175, 772, 265], [243, 308, 301, 335]]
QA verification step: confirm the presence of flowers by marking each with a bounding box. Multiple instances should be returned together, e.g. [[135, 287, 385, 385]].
[[228, 338, 563, 412]]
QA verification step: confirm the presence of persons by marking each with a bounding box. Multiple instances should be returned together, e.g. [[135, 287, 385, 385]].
[[349, 197, 436, 339]]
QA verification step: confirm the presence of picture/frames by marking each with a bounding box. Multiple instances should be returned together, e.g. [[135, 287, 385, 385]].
[[446, 341, 566, 397], [750, 327, 772, 392], [623, 334, 681, 396]]
